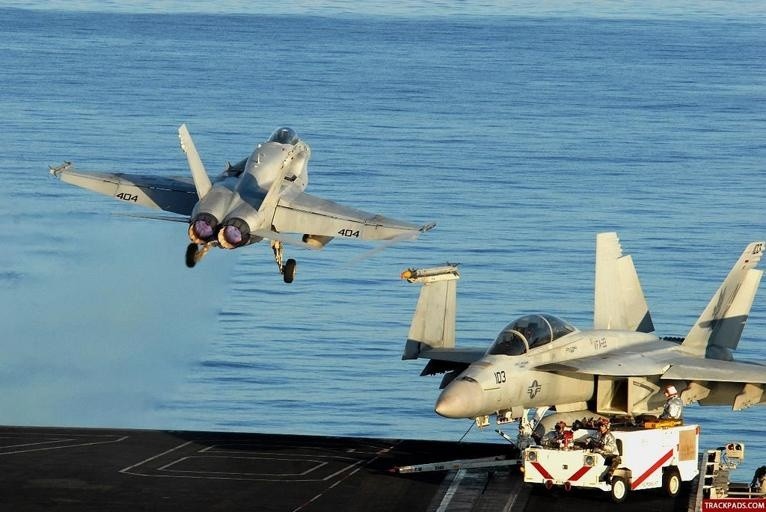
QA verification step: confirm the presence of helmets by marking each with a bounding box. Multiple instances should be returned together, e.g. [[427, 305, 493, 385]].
[[597, 418, 608, 426], [555, 421, 565, 428], [663, 384, 677, 396]]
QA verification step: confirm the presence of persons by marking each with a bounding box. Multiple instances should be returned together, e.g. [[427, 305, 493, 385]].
[[586, 418, 621, 482], [658, 384, 685, 418], [541, 421, 567, 446]]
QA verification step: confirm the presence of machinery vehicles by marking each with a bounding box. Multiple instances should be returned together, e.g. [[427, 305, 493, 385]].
[[520, 424, 700, 504]]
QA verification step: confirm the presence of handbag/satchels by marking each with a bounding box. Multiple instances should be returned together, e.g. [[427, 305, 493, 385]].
[[589, 441, 600, 448]]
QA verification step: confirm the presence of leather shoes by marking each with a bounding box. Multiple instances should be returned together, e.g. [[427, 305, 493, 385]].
[[604, 475, 612, 484]]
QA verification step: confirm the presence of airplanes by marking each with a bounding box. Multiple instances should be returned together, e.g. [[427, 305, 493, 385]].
[[46, 122, 437, 284], [400, 230, 765, 459]]
